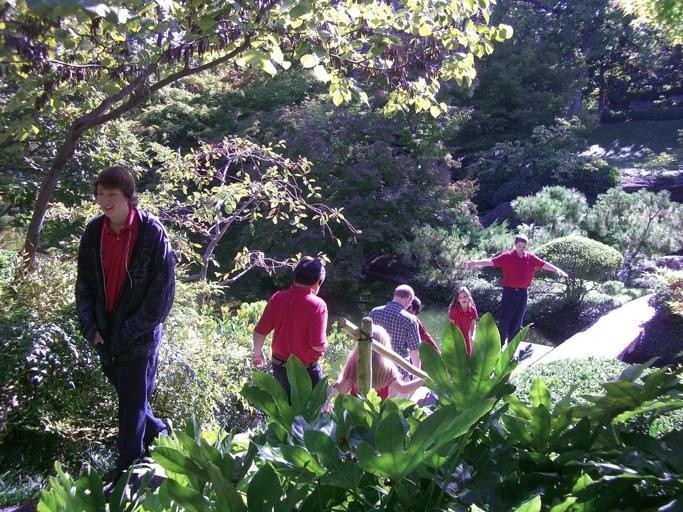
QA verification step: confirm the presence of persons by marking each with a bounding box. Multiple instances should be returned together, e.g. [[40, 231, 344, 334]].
[[332, 323, 425, 401], [251, 256, 330, 405], [463, 234, 569, 349], [405, 294, 442, 354], [73, 167, 174, 464], [446, 284, 479, 361], [365, 284, 421, 401]]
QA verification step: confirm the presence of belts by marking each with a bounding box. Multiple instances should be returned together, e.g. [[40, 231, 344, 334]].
[[505, 287, 527, 291]]
